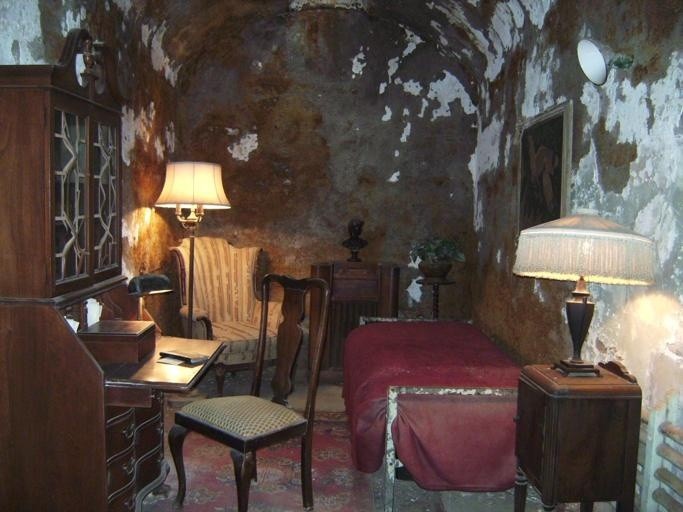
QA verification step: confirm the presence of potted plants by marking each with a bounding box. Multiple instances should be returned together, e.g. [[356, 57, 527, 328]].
[[410, 235, 459, 278]]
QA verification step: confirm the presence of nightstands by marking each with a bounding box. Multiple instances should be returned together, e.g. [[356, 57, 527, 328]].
[[513, 362, 641, 510]]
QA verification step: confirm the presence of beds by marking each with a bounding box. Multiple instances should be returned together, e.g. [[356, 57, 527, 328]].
[[349, 315, 533, 512]]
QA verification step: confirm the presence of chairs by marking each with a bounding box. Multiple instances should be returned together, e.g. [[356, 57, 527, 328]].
[[162, 234, 284, 399], [164, 273, 331, 511]]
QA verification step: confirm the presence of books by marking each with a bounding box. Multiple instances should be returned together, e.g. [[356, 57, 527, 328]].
[[160, 349, 209, 365]]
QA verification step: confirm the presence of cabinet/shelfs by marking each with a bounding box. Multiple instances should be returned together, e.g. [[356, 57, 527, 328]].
[[0, 30, 172, 510]]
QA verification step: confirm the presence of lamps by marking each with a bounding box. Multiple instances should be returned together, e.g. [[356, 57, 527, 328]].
[[509, 214, 656, 377], [153, 158, 230, 339]]
